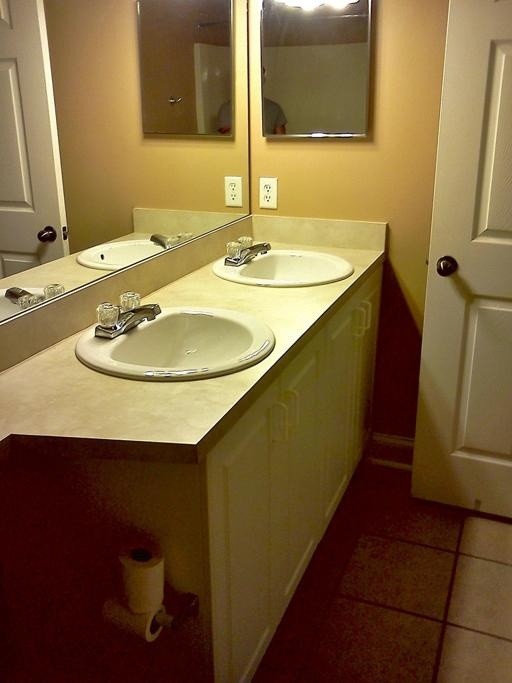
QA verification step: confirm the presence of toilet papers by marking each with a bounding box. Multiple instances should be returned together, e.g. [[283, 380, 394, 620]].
[[104, 598, 166, 642], [116, 540, 167, 613]]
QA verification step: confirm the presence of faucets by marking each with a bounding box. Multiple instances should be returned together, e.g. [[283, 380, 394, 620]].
[[4, 287, 36, 307], [151, 233, 173, 248], [118, 303, 162, 332], [241, 243, 271, 258]]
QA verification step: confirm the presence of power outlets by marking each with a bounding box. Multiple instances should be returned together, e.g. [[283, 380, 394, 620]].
[[224, 175, 243, 208], [258, 176, 278, 210]]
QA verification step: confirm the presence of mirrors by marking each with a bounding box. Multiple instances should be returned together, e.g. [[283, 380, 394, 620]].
[[1, 0, 254, 326], [257, 0, 376, 140], [135, 1, 237, 140]]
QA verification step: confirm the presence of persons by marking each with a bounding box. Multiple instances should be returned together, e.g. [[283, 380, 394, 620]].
[[216, 66, 287, 136]]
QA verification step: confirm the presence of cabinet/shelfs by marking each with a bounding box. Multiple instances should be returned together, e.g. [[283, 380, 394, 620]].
[[0, 256, 384, 682]]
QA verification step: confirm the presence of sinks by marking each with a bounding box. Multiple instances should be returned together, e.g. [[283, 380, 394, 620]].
[[75, 306, 275, 379], [211, 248, 354, 287], [77, 241, 163, 270], [1, 284, 44, 322]]
